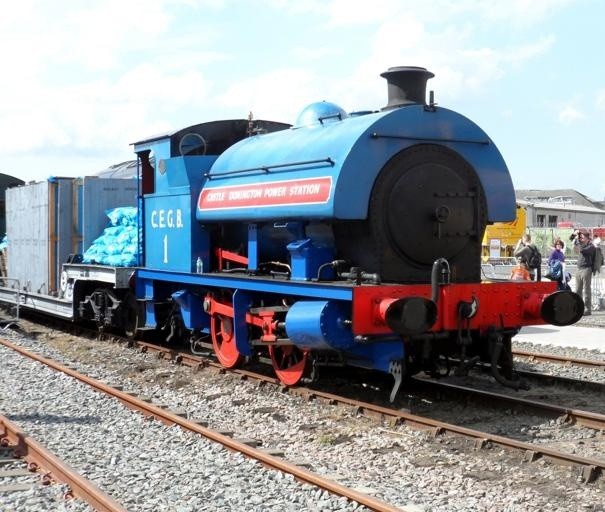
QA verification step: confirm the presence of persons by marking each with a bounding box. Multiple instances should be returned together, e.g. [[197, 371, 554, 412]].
[[593, 234, 601, 247], [570, 229, 596, 316], [513, 233, 541, 280], [548, 240, 565, 289]]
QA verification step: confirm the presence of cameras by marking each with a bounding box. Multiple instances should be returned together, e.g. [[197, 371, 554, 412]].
[[569, 231, 582, 241], [520, 239, 523, 244]]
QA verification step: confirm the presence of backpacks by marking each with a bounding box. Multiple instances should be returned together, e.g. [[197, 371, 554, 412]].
[[523, 245, 541, 268], [589, 242, 604, 276]]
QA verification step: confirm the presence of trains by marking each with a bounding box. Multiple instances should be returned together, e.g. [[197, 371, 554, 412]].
[[0, 64, 585, 403]]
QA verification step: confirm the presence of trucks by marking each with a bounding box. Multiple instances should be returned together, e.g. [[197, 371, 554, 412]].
[[556, 222, 605, 239], [480, 204, 527, 263]]
